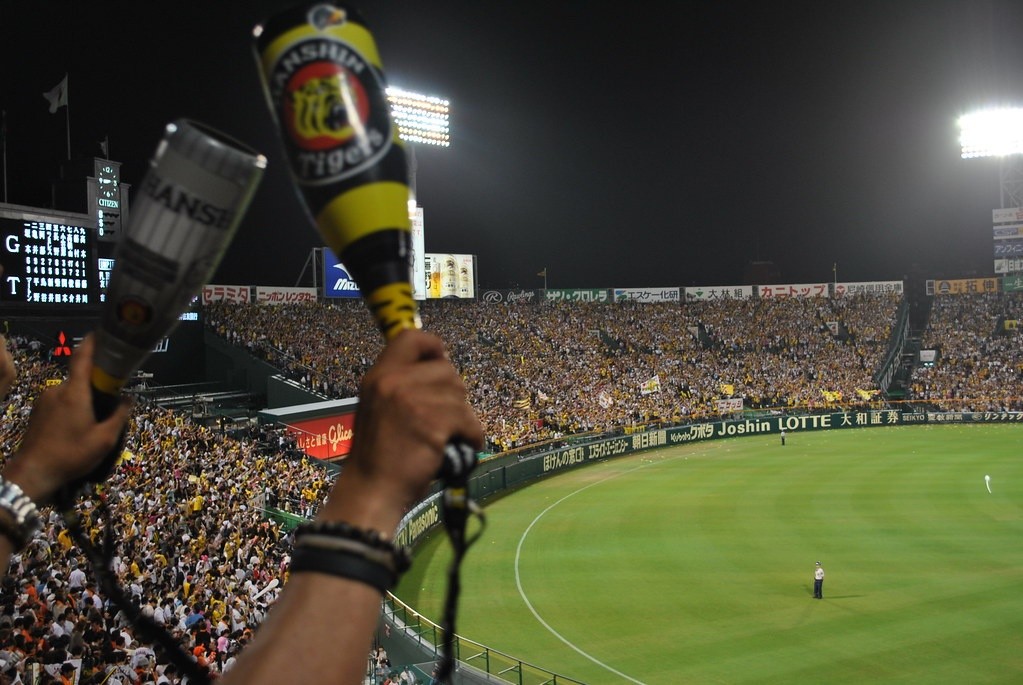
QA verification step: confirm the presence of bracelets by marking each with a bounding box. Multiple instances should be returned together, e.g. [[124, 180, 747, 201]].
[[294, 520, 413, 575], [0, 475, 43, 536], [0, 519, 27, 554], [287, 548, 393, 598]]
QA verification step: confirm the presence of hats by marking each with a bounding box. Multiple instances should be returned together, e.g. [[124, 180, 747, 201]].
[[123, 555, 130, 561], [138, 657, 150, 666], [194, 643, 206, 656], [19, 578, 32, 587], [62, 662, 78, 671], [187, 597, 192, 603]]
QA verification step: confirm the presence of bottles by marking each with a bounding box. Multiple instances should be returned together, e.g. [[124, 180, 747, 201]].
[[88, 117, 268, 428], [253, 0, 423, 342]]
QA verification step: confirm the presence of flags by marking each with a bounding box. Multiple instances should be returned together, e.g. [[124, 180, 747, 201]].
[[43, 75, 68, 113]]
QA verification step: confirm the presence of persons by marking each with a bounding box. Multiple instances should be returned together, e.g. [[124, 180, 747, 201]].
[[0, 328, 486, 685], [207, 290, 903, 453], [781, 429, 786, 445], [813, 561, 824, 599], [0, 332, 436, 685], [909, 291, 1023, 412]]
